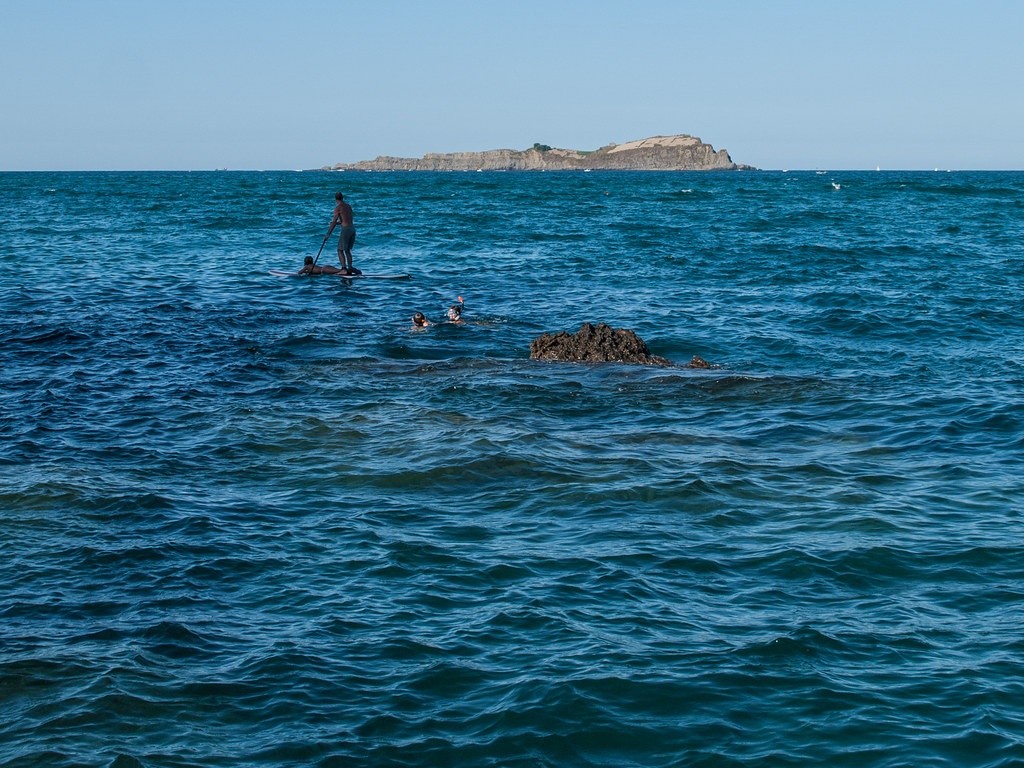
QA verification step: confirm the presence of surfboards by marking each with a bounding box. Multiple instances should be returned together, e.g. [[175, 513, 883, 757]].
[[269, 269, 408, 278]]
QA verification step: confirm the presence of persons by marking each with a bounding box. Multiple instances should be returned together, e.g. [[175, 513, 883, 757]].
[[445, 306, 498, 332], [299, 256, 363, 275], [401, 312, 437, 335], [323, 192, 356, 276]]
